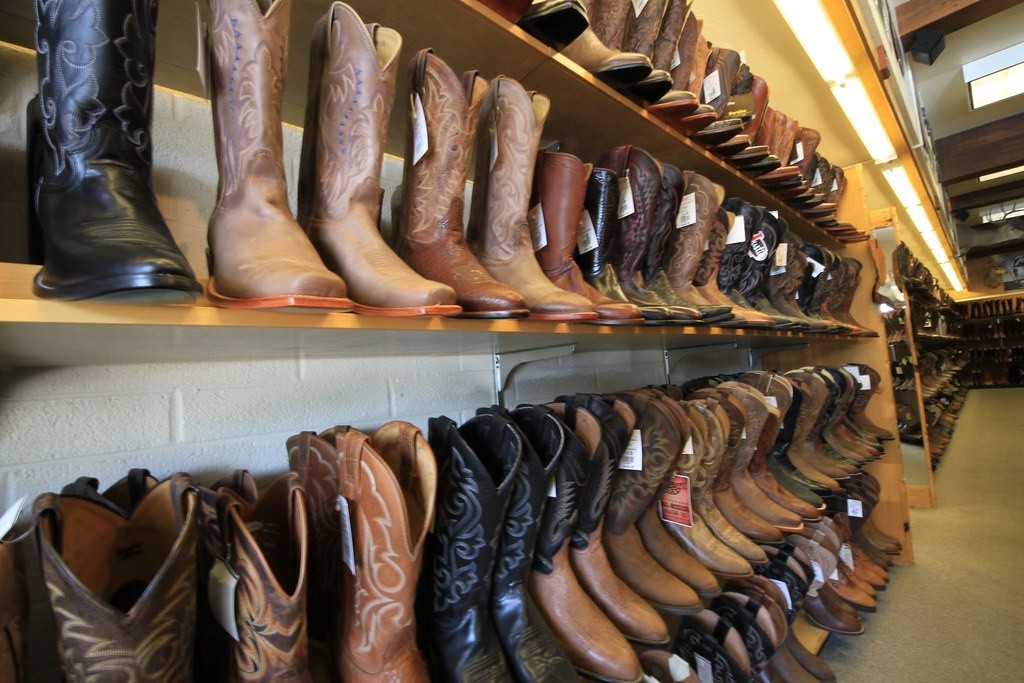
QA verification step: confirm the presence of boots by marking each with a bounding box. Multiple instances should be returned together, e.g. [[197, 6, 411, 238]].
[[26, 0, 881, 335], [0, 363, 903, 683], [883, 241, 1024, 472]]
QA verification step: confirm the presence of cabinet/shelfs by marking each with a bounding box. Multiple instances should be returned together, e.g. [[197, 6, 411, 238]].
[[0, 2, 1023, 683]]
